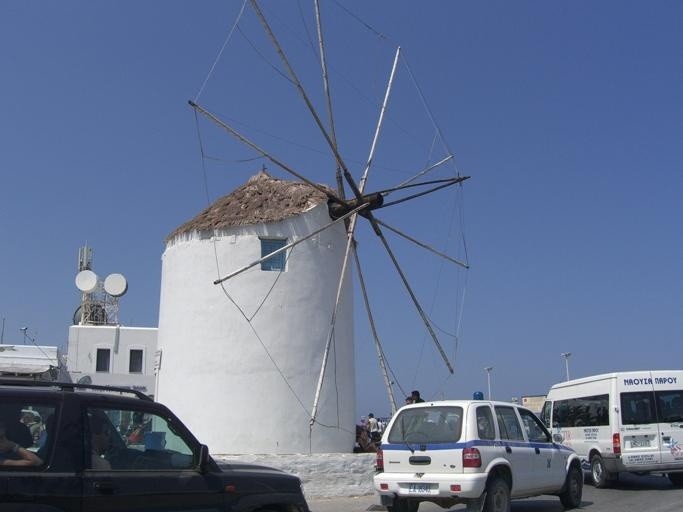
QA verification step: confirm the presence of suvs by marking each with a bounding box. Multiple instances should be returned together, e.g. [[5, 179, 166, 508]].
[[1, 379, 311, 511]]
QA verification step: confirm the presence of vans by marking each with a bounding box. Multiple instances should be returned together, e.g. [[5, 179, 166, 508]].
[[539, 369, 683, 489]]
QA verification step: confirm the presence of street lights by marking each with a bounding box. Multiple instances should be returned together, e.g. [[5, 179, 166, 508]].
[[483, 366, 493, 400], [561, 351, 573, 382]]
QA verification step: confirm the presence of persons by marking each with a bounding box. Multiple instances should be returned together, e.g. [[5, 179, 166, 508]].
[[87, 413, 114, 470], [112, 411, 151, 446], [405, 396, 413, 405], [0, 413, 45, 468], [12, 410, 55, 447], [411, 391, 425, 403], [357, 413, 386, 441]]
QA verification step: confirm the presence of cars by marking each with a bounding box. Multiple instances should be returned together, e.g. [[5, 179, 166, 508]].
[[373, 391, 583, 512]]
